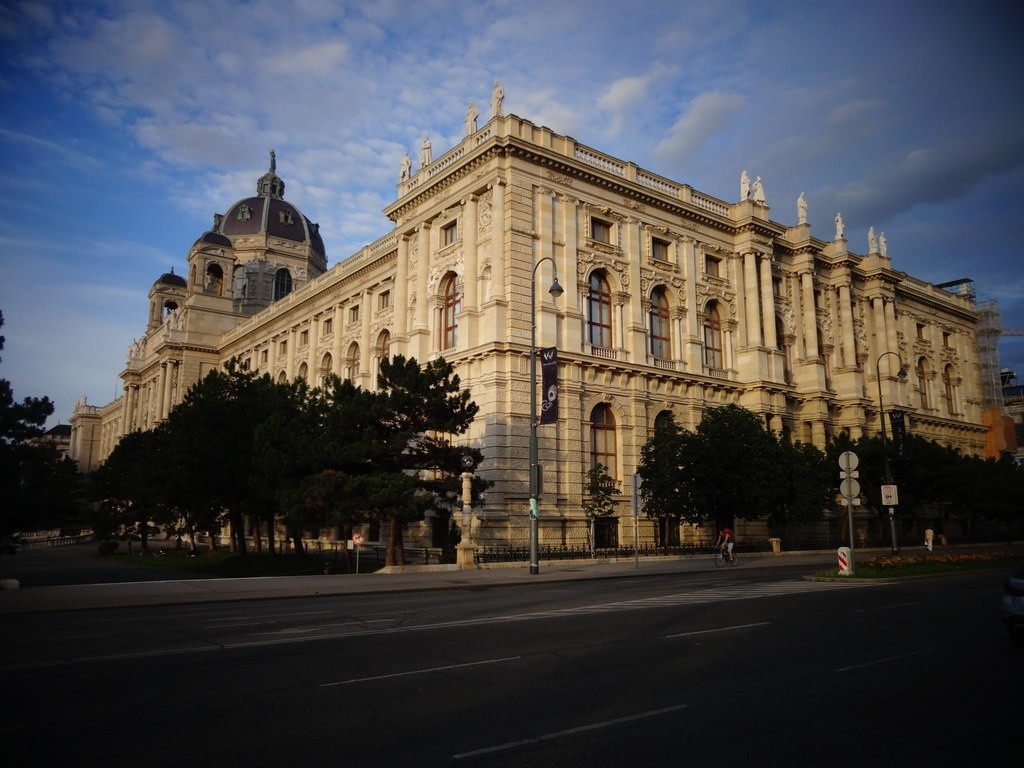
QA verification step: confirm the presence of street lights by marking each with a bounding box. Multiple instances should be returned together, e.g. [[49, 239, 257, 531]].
[[527, 257, 565, 576], [876, 351, 909, 556]]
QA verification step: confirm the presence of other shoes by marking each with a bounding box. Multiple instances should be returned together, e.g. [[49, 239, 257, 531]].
[[730, 558, 733, 560]]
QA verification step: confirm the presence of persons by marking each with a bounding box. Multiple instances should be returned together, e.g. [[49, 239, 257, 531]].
[[464, 102, 479, 135], [269, 148, 276, 173], [426, 258, 464, 298], [714, 531, 734, 561], [400, 151, 411, 183], [256, 176, 285, 199], [740, 170, 765, 205], [128, 336, 147, 361], [279, 207, 292, 225], [163, 306, 186, 331], [419, 136, 431, 167], [73, 394, 87, 412], [490, 81, 504, 115], [834, 213, 845, 238], [206, 272, 214, 292], [797, 192, 808, 224], [925, 526, 934, 551], [868, 226, 887, 258], [238, 204, 250, 219]]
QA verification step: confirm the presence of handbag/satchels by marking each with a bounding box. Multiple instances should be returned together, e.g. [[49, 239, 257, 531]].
[[925, 540, 928, 546]]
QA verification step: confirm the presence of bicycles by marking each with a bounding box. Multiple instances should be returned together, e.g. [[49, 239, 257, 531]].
[[713, 545, 738, 569]]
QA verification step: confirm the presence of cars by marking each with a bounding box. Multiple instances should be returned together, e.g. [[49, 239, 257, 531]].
[[1001, 559, 1024, 648]]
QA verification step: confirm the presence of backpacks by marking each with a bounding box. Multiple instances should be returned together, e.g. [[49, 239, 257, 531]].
[[723, 528, 735, 542]]
